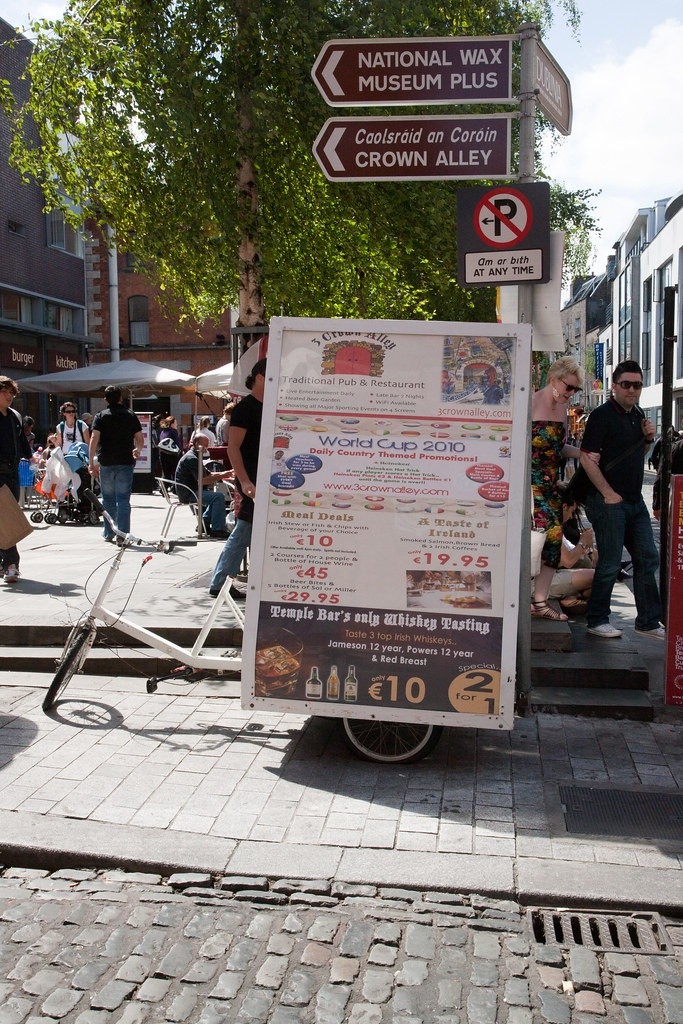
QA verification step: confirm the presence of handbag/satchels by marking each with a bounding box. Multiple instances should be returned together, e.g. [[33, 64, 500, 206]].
[[158, 438, 180, 455], [531, 529, 548, 576], [565, 469, 594, 506], [0, 484, 32, 549]]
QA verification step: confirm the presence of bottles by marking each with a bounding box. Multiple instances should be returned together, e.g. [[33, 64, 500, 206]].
[[306, 666, 322, 699], [33, 446, 44, 463], [327, 665, 340, 700], [343, 665, 358, 702]]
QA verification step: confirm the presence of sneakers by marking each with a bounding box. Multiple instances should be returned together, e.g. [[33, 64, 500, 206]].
[[0, 561, 4, 578], [3, 564, 20, 583], [634, 627, 666, 639], [586, 624, 622, 637]]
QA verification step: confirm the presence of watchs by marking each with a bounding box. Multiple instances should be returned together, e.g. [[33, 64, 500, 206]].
[[645, 437, 656, 443]]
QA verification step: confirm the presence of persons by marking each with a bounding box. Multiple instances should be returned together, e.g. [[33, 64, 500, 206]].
[[530, 425, 683, 606], [0, 374, 31, 584], [19, 401, 240, 539], [209, 357, 267, 598], [88, 385, 144, 544], [532, 355, 601, 619], [579, 360, 665, 641]]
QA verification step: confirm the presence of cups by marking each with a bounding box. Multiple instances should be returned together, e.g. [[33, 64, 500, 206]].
[[255, 646, 299, 697]]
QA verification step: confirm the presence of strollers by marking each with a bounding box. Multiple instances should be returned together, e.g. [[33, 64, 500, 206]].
[[29, 441, 100, 527]]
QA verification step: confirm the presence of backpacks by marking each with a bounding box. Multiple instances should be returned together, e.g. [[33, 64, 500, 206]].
[[648, 430, 679, 467]]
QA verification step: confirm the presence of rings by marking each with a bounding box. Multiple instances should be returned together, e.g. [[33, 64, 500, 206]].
[[247, 491, 251, 494]]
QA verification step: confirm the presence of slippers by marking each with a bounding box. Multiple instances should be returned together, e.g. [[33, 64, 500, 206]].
[[560, 600, 588, 615]]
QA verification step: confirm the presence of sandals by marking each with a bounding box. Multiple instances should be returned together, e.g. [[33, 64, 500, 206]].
[[531, 600, 568, 621]]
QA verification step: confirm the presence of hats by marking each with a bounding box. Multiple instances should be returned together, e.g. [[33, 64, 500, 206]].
[[81, 413, 93, 420]]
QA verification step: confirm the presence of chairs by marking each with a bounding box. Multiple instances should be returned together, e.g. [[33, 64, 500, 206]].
[[154, 476, 208, 540]]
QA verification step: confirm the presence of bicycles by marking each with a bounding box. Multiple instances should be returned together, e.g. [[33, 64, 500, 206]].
[[41, 485, 442, 770]]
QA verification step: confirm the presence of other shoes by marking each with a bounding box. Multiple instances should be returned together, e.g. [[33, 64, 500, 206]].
[[210, 586, 245, 598], [116, 536, 130, 547], [621, 560, 633, 572], [104, 537, 112, 542], [618, 569, 633, 581], [210, 530, 230, 537], [195, 527, 211, 533]]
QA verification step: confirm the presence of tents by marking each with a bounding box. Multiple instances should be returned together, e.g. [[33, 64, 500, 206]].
[[14, 357, 234, 427]]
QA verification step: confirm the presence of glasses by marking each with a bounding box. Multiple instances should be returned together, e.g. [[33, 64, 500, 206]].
[[65, 410, 76, 413], [560, 379, 579, 394], [616, 381, 643, 389]]
